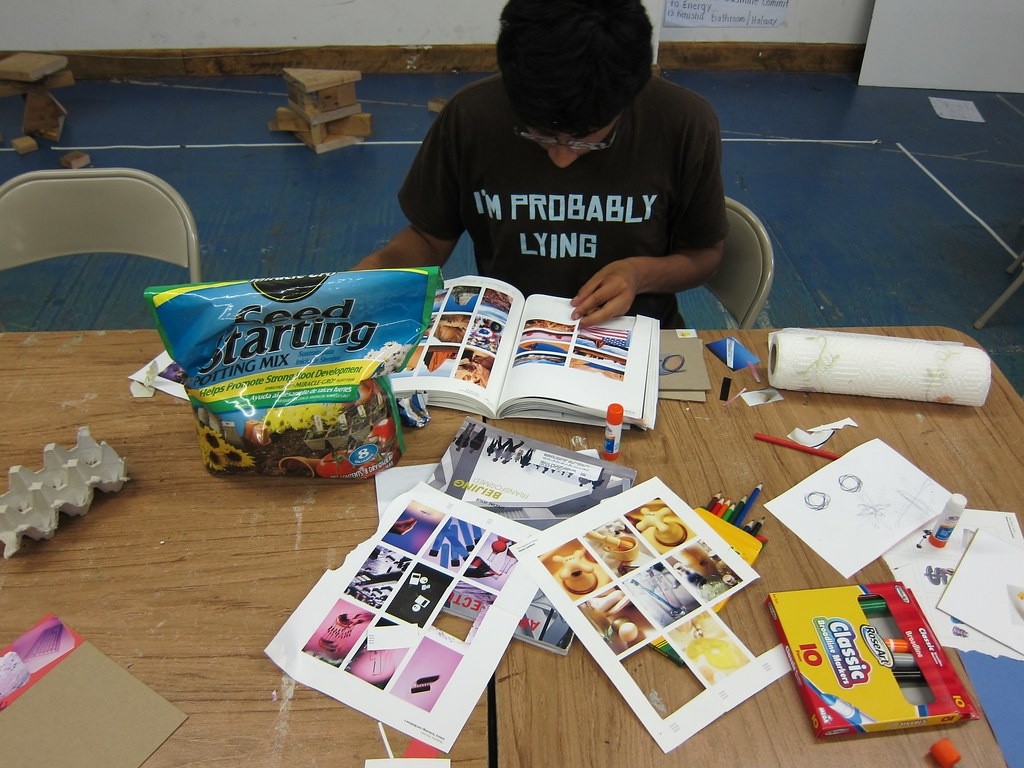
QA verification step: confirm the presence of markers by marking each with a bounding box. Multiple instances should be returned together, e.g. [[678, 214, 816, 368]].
[[858, 593, 925, 680]]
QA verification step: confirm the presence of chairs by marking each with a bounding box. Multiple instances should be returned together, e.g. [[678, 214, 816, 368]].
[[702, 195, 775, 329], [0, 166, 204, 284]]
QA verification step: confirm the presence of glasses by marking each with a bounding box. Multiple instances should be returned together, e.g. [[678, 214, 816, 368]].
[[512, 120, 620, 149]]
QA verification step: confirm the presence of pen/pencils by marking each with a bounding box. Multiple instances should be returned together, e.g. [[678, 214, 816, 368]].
[[705, 481, 766, 536], [753, 432, 841, 460]]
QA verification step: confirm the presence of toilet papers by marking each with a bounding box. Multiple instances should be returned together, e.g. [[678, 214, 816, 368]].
[[766, 327, 991, 407]]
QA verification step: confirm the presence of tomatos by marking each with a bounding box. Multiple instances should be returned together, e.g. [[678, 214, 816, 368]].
[[314, 416, 400, 478]]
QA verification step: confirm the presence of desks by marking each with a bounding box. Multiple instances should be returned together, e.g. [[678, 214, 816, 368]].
[[0, 331, 491, 768], [489, 327, 1024, 768]]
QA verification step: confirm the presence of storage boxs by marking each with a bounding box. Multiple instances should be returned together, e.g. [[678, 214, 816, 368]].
[[764, 581, 981, 741]]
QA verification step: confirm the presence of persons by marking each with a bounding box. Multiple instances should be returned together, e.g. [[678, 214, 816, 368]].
[[455, 422, 606, 490], [351, 1, 727, 329]]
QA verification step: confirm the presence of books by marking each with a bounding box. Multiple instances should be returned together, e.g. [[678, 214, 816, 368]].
[[390, 275, 659, 431], [426, 415, 639, 656]]
